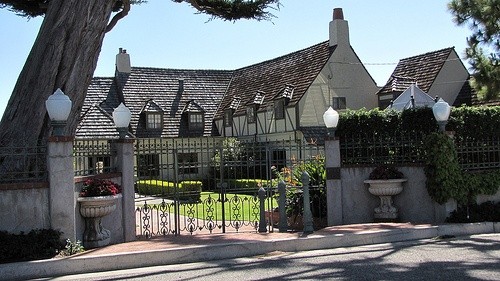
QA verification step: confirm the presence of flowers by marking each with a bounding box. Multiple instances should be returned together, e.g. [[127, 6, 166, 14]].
[[79, 175, 124, 197], [369, 163, 404, 181]]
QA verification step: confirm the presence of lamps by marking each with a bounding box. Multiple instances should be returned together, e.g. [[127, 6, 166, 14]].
[[432, 97, 451, 135], [322, 105, 341, 141], [45, 87, 73, 137], [112, 103, 133, 139]]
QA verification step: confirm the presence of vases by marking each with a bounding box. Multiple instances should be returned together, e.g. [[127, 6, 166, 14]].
[[363, 178, 409, 219], [77, 192, 123, 248]]
